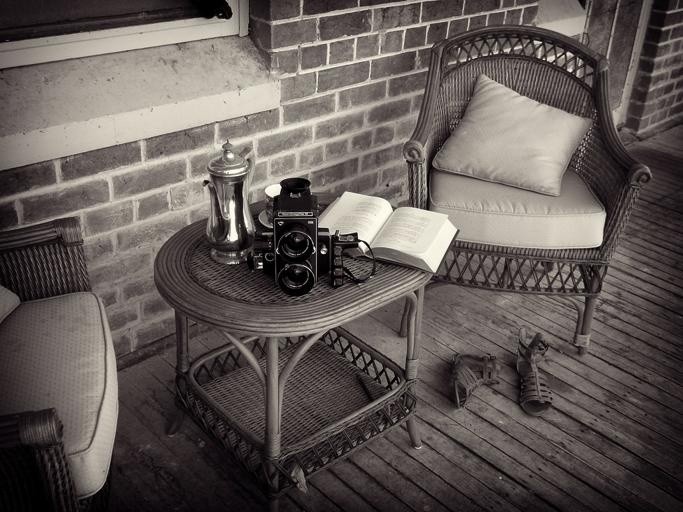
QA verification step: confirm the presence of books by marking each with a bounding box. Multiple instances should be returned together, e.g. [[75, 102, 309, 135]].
[[315, 188, 461, 275]]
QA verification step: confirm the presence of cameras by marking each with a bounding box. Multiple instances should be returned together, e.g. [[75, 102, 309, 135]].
[[248, 178, 360, 298]]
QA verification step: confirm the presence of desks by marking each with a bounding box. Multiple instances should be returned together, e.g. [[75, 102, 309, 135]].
[[153, 208, 434, 512]]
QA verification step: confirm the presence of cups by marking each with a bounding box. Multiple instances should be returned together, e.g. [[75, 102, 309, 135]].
[[280, 178, 312, 213], [264, 185, 282, 216]]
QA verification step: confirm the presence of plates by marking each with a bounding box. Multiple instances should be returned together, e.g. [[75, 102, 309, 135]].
[[258, 210, 274, 230]]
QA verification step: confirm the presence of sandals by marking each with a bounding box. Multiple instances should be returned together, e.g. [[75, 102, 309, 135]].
[[513, 322, 559, 419], [447, 347, 502, 411]]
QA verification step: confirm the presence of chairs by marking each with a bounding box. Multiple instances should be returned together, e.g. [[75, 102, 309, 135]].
[[400, 27, 653, 358], [0, 215, 117, 511]]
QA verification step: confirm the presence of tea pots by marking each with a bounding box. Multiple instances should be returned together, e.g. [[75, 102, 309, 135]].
[[202, 138, 255, 265]]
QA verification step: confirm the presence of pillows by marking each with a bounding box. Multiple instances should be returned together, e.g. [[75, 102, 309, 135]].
[[427, 72, 596, 198]]
